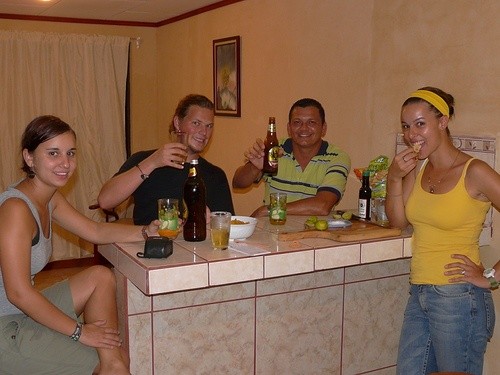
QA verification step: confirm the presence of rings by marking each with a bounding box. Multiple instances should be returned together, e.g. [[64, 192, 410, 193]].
[[462, 270, 465, 275]]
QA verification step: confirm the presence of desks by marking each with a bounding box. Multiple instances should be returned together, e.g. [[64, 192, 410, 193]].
[[98, 213, 412, 375]]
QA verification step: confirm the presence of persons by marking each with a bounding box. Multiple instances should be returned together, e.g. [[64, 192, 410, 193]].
[[97, 93, 235, 225], [384, 87, 500, 375], [232, 97, 352, 218], [0, 114, 182, 375]]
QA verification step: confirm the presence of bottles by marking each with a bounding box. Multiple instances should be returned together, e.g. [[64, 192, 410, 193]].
[[358, 170, 372, 221], [182, 159, 207, 242], [262, 117, 278, 176]]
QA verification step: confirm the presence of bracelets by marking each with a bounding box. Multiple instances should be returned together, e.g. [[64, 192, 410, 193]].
[[138, 165, 146, 181], [142, 225, 148, 239], [71, 322, 82, 341], [386, 193, 404, 197]]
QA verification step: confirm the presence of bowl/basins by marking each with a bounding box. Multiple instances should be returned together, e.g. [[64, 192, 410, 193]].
[[228, 215, 258, 242]]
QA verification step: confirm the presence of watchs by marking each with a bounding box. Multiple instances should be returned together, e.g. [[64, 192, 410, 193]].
[[482, 268, 498, 290]]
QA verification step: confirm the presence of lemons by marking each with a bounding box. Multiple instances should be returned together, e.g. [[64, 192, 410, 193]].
[[333, 212, 352, 220], [304, 216, 328, 230]]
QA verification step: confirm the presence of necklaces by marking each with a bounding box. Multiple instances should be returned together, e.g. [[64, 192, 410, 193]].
[[428, 150, 459, 193]]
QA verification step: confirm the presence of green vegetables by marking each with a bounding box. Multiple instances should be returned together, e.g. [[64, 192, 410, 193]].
[[269, 204, 286, 220], [367, 155, 387, 197], [158, 206, 178, 231]]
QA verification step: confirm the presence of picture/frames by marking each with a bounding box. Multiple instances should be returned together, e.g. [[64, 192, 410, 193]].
[[213, 36, 240, 116]]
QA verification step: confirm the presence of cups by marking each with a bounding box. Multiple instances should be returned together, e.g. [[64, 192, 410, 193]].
[[171, 131, 188, 164], [210, 211, 231, 250], [374, 198, 389, 226], [158, 199, 179, 241], [270, 193, 287, 225]]
